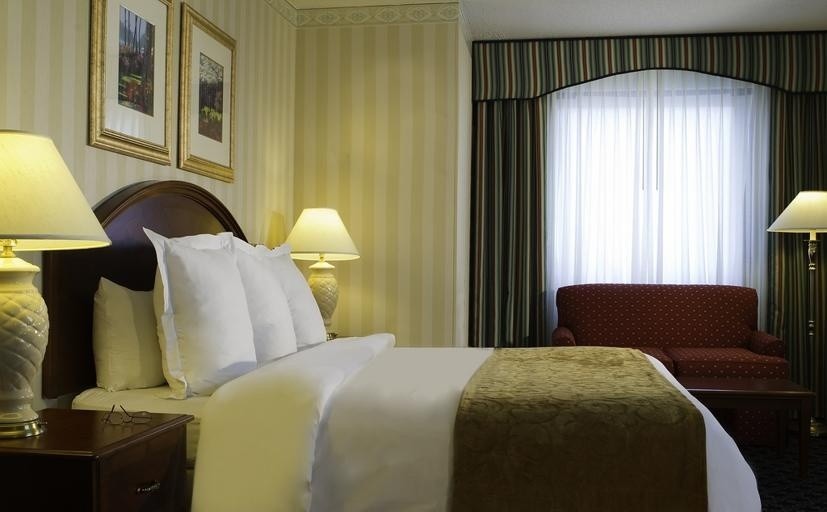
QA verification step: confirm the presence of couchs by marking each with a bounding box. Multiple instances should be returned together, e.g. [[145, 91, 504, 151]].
[[552, 284, 792, 447]]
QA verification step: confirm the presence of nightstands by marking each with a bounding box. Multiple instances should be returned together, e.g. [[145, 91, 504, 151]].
[[0, 409, 194, 512]]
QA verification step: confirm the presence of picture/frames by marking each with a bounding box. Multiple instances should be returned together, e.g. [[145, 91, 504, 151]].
[[179, 2, 236, 181], [89, 1, 171, 165]]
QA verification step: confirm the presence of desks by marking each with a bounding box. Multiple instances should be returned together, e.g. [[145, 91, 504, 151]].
[[673, 377, 815, 478]]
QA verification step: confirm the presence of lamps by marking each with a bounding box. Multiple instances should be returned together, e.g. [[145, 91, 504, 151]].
[[766, 190, 826, 438], [1, 130, 111, 440], [283, 206, 360, 339]]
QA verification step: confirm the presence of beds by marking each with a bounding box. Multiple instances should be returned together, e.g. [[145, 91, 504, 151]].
[[42, 180, 763, 512]]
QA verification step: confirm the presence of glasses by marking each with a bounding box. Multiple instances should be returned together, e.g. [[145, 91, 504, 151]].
[[102, 405, 152, 424]]
